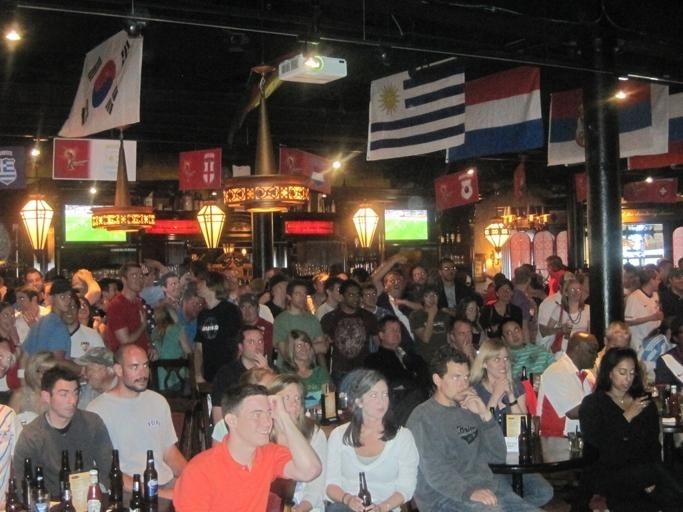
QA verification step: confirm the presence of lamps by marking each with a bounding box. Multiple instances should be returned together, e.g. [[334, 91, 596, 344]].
[[643, 388, 683, 476], [485, 203, 515, 255], [606, 57, 636, 85], [18, 199, 54, 251], [352, 208, 379, 248], [293, 19, 324, 69], [195, 204, 226, 248]]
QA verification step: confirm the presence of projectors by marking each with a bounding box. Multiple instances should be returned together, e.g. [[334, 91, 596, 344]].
[[277, 45, 348, 85]]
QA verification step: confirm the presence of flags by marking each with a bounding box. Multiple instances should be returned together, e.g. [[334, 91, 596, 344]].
[[57, 29, 142, 138], [445, 64, 544, 164], [624, 91, 683, 169], [547, 81, 669, 170], [365, 55, 467, 166]]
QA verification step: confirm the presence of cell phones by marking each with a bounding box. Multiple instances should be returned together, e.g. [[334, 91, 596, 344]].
[[641, 390, 653, 405]]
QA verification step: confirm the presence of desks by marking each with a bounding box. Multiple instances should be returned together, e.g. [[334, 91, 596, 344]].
[[484, 434, 601, 497]]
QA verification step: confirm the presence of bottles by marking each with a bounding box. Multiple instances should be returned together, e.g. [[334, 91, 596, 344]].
[[22, 456, 37, 511], [668, 385, 680, 429], [145, 449, 159, 512], [86, 469, 105, 512], [520, 367, 528, 382], [108, 450, 123, 501], [358, 470, 372, 510], [129, 474, 145, 511], [519, 415, 532, 466], [74, 449, 85, 475], [59, 450, 74, 502], [4, 477, 24, 512], [32, 471, 50, 512], [59, 482, 76, 512]]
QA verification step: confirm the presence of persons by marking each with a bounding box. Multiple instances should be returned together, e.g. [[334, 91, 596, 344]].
[[268, 374, 327, 512], [511, 266, 537, 344], [590, 321, 648, 387], [409, 286, 455, 366], [315, 276, 343, 320], [160, 272, 182, 324], [480, 282, 523, 337], [2, 268, 118, 359], [223, 268, 288, 324], [193, 271, 244, 391], [661, 268, 682, 331], [237, 293, 272, 358], [0, 401, 23, 512], [579, 347, 683, 512], [377, 271, 421, 341], [9, 349, 55, 419], [404, 348, 545, 512], [139, 265, 163, 306], [0, 337, 11, 404], [350, 267, 368, 285], [360, 283, 392, 318], [446, 296, 485, 348], [364, 314, 426, 394], [85, 344, 188, 500], [106, 262, 149, 357], [76, 347, 117, 411], [469, 338, 522, 428], [14, 365, 114, 503], [431, 257, 465, 317], [320, 279, 379, 382], [404, 265, 429, 303], [623, 258, 683, 298], [173, 384, 321, 512], [535, 331, 598, 463], [325, 370, 420, 512], [212, 326, 275, 424], [311, 272, 329, 306], [447, 317, 475, 362], [273, 281, 329, 370], [501, 318, 553, 377], [625, 268, 664, 355], [151, 302, 192, 392], [538, 255, 591, 353]]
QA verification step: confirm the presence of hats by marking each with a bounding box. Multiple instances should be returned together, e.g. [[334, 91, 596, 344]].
[[492, 273, 504, 291], [50, 279, 79, 298], [240, 292, 258, 310], [669, 317, 683, 344], [99, 277, 123, 292], [270, 275, 287, 289], [72, 347, 114, 367]]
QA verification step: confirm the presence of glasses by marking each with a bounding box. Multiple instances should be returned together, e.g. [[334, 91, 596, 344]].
[[673, 330, 682, 334], [441, 266, 456, 271]]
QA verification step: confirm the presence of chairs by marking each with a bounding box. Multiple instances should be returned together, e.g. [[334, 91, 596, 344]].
[[165, 397, 199, 459], [150, 353, 194, 396], [197, 382, 215, 450]]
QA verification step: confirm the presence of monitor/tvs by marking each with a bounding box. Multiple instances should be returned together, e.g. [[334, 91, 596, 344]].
[[60, 200, 133, 249], [379, 206, 431, 247]]
